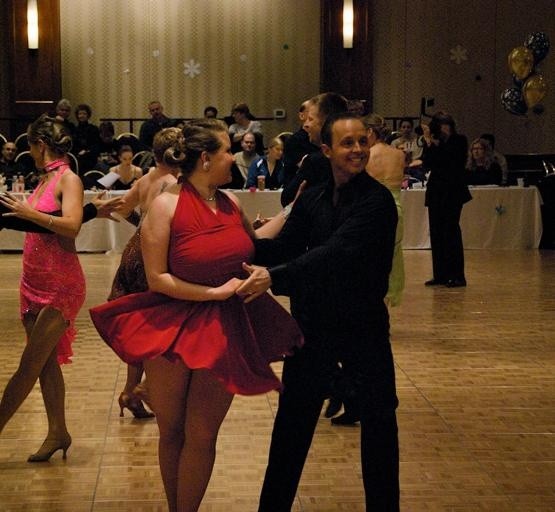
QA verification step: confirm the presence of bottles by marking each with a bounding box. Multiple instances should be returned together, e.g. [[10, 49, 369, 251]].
[[10, 175, 24, 193]]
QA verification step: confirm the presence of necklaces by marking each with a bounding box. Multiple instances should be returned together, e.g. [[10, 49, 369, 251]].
[[202, 197, 217, 201]]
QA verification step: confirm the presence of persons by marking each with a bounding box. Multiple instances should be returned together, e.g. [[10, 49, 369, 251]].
[[55, 99, 263, 188], [279, 99, 316, 178], [234, 113, 401, 512], [0, 190, 127, 235], [106, 126, 263, 420], [280, 93, 362, 437], [0, 141, 25, 191], [364, 113, 404, 309], [0, 115, 88, 463], [424, 111, 474, 286], [89, 119, 307, 511], [390, 118, 506, 186]]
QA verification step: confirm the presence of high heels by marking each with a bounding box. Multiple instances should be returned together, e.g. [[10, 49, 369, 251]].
[[28, 437, 71, 462], [118, 390, 155, 419]]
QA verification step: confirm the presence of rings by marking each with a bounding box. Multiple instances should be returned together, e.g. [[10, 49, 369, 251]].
[[249, 289, 253, 295]]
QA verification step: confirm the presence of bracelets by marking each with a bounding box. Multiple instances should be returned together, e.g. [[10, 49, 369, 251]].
[[280, 204, 294, 220], [46, 213, 54, 231]]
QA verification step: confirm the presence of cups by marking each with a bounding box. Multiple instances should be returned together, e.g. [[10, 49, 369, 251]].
[[93, 186, 97, 192], [249, 175, 267, 194], [517, 178, 525, 189], [101, 191, 112, 201]]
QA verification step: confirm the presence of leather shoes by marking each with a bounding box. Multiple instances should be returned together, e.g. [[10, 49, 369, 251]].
[[424, 279, 466, 287], [324, 398, 342, 418], [331, 412, 361, 426]]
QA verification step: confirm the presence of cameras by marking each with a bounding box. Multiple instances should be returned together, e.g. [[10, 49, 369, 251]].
[[414, 95, 443, 142]]
